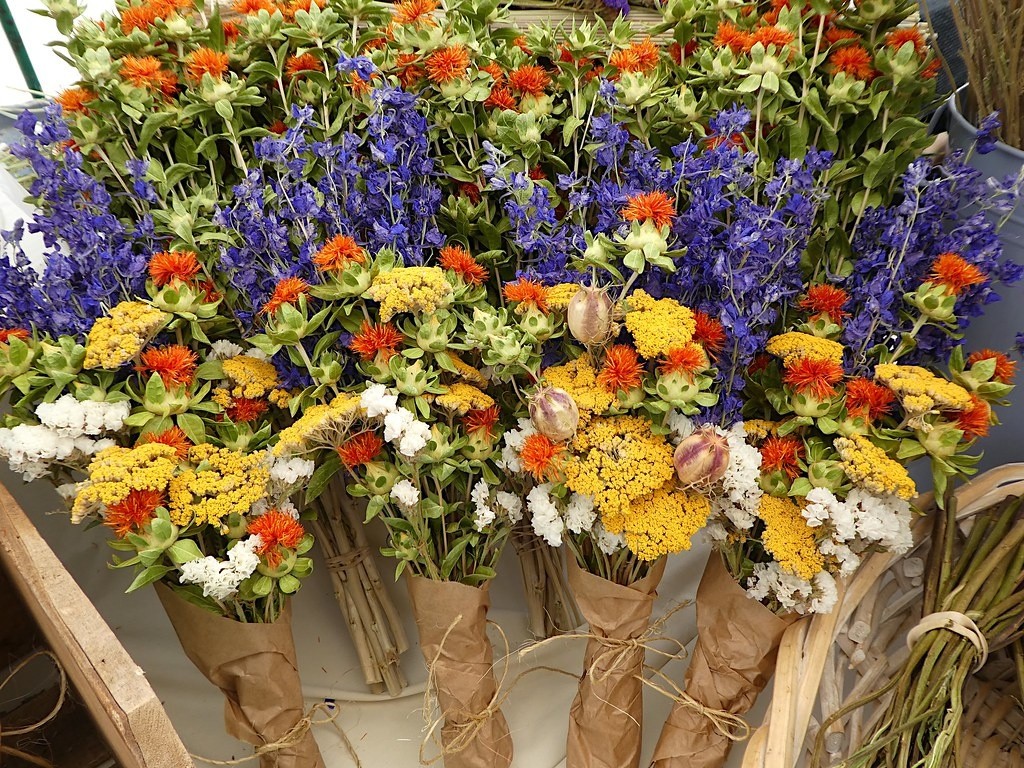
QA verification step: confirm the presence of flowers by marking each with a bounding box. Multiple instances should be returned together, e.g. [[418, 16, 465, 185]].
[[0, 1, 1023, 768]]
